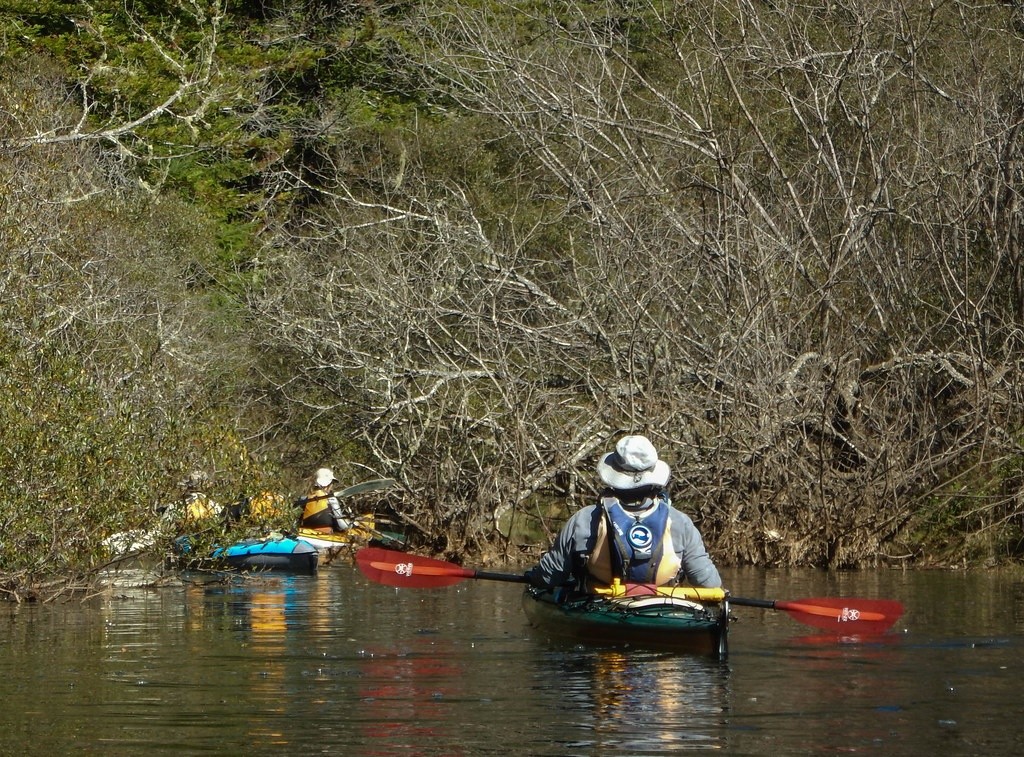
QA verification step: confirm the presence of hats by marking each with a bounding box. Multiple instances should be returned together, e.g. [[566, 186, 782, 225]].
[[314, 469, 339, 486], [598, 435, 670, 489], [180, 470, 213, 491]]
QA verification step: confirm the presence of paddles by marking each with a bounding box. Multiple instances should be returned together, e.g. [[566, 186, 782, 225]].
[[291, 476, 397, 509], [356, 522, 406, 547], [352, 543, 904, 636]]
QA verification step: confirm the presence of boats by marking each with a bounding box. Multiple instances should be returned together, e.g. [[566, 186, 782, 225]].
[[298, 514, 375, 556], [166, 533, 319, 574], [522, 569, 730, 659]]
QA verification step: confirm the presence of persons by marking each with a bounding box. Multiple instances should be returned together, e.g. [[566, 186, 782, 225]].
[[292, 468, 355, 532], [525, 436, 731, 604], [153, 471, 287, 537]]
